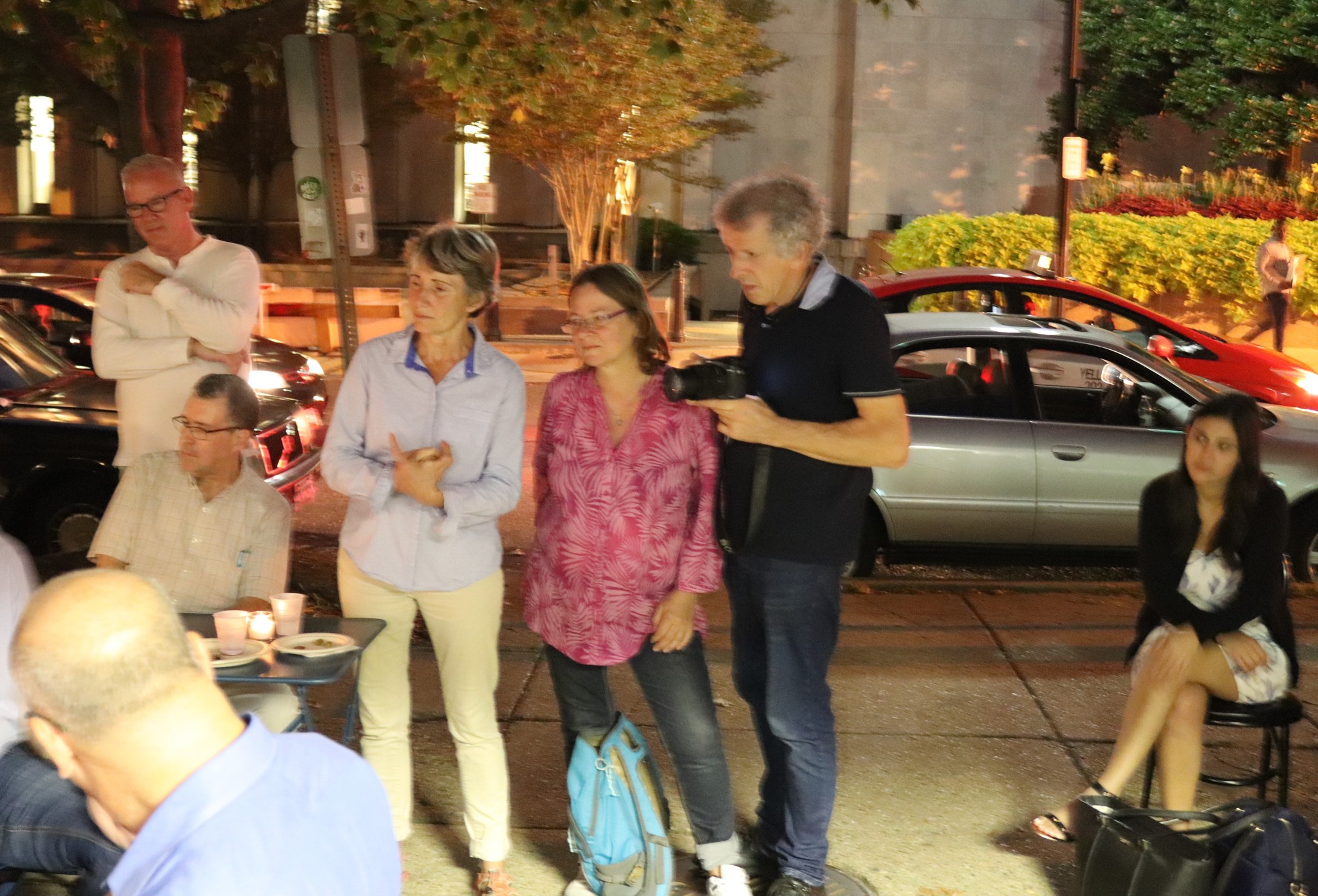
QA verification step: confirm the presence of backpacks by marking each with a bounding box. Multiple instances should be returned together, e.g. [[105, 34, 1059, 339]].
[[564, 711, 675, 896]]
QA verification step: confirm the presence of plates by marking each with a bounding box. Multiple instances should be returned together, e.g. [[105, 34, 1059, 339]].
[[203, 638, 269, 668], [270, 632, 354, 655]]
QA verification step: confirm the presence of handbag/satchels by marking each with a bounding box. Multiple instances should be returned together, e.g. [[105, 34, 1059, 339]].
[[1073, 794, 1277, 896], [1203, 802, 1318, 896]]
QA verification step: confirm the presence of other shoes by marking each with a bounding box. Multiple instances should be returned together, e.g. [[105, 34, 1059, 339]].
[[693, 842, 826, 896]]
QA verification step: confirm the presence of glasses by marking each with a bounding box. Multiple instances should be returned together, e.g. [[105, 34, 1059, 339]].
[[172, 414, 251, 440], [121, 188, 183, 219], [559, 308, 631, 335]]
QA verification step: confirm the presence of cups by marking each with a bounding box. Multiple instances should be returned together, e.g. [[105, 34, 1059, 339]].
[[269, 593, 304, 636], [213, 610, 250, 655]]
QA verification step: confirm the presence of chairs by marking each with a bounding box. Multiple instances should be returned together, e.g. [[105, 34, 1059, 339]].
[[1137, 596, 1306, 812], [945, 356, 972, 385], [980, 358, 1008, 388]]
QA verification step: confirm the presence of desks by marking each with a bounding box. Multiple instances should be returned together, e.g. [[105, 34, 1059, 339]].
[[175, 612, 389, 748]]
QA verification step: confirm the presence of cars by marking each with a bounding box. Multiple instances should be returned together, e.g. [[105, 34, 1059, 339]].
[[0, 308, 122, 561], [721, 316, 1318, 583], [6, 273, 331, 504], [858, 266, 1318, 413]]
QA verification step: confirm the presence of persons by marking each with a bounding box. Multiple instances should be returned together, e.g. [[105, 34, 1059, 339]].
[[88, 375, 289, 641], [518, 265, 752, 896], [13, 571, 403, 896], [681, 173, 909, 896], [1030, 393, 1301, 845], [0, 539, 125, 896], [321, 218, 527, 896], [91, 153, 262, 480], [1239, 218, 1297, 353]]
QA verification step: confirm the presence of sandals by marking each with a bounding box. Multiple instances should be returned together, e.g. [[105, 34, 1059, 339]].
[[1028, 812, 1078, 843]]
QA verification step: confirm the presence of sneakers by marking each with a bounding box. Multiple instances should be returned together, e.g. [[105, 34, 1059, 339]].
[[564, 864, 596, 896], [473, 868, 519, 896], [706, 864, 753, 896]]
